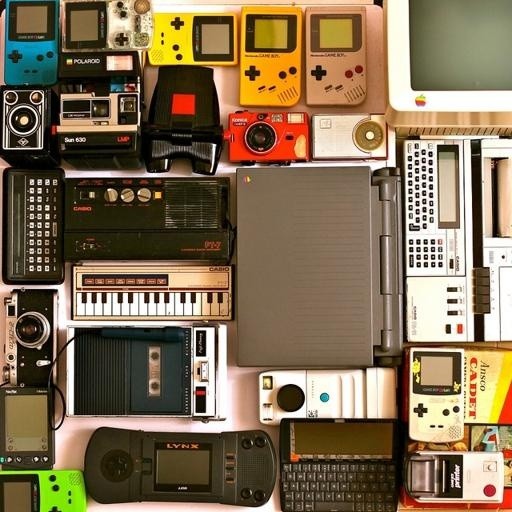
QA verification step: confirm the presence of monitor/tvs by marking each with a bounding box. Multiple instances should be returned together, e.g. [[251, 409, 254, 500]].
[[384, 0, 512, 113]]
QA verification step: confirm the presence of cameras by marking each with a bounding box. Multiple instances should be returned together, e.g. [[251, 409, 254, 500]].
[[228, 113, 311, 163], [4, 288, 58, 386], [312, 112, 389, 161]]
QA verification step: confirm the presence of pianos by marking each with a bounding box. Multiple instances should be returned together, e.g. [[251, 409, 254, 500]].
[[70, 261, 234, 323]]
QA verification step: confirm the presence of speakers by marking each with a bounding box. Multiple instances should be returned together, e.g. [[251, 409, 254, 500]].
[[0, 85, 60, 168]]
